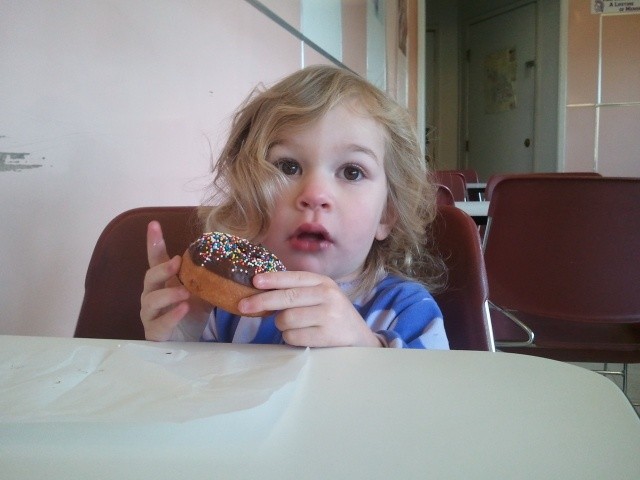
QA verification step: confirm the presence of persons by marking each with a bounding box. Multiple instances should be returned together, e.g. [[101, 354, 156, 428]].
[[137, 62, 452, 350]]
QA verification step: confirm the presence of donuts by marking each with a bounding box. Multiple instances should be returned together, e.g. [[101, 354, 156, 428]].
[[179, 232, 289, 318]]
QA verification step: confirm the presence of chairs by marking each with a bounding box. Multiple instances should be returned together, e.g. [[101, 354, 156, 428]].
[[67, 198, 495, 349], [480, 175, 640, 412], [428, 175, 465, 201], [430, 183, 455, 206]]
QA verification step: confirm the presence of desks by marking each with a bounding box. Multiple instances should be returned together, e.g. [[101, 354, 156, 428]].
[[0, 330, 640, 480], [454, 199, 491, 222]]
